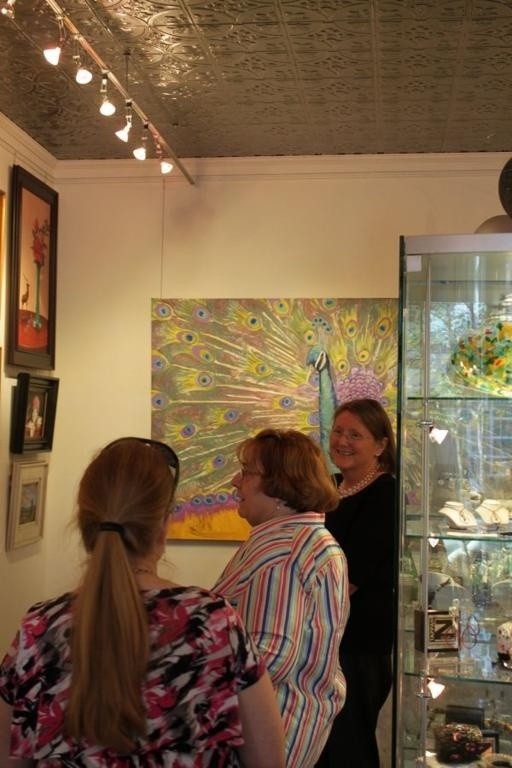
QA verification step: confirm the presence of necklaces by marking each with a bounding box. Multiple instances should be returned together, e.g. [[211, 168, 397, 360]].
[[338, 465, 378, 499]]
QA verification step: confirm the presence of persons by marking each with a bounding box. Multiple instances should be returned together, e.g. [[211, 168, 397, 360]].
[[0, 436, 288, 768], [315, 398, 397, 768], [204, 427, 352, 768], [23, 396, 43, 439]]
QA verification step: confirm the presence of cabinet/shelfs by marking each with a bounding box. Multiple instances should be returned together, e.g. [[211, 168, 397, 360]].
[[390, 232, 512, 768]]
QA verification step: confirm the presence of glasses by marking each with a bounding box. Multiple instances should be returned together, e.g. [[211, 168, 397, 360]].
[[332, 428, 374, 441], [243, 468, 262, 480], [104, 436, 180, 515]]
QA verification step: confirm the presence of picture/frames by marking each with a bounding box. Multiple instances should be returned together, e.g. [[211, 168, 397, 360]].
[[7, 163, 59, 371], [7, 459, 48, 552], [10, 371, 60, 455]]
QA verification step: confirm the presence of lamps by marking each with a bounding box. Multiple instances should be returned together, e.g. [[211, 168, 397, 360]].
[[0, 0, 194, 188]]
